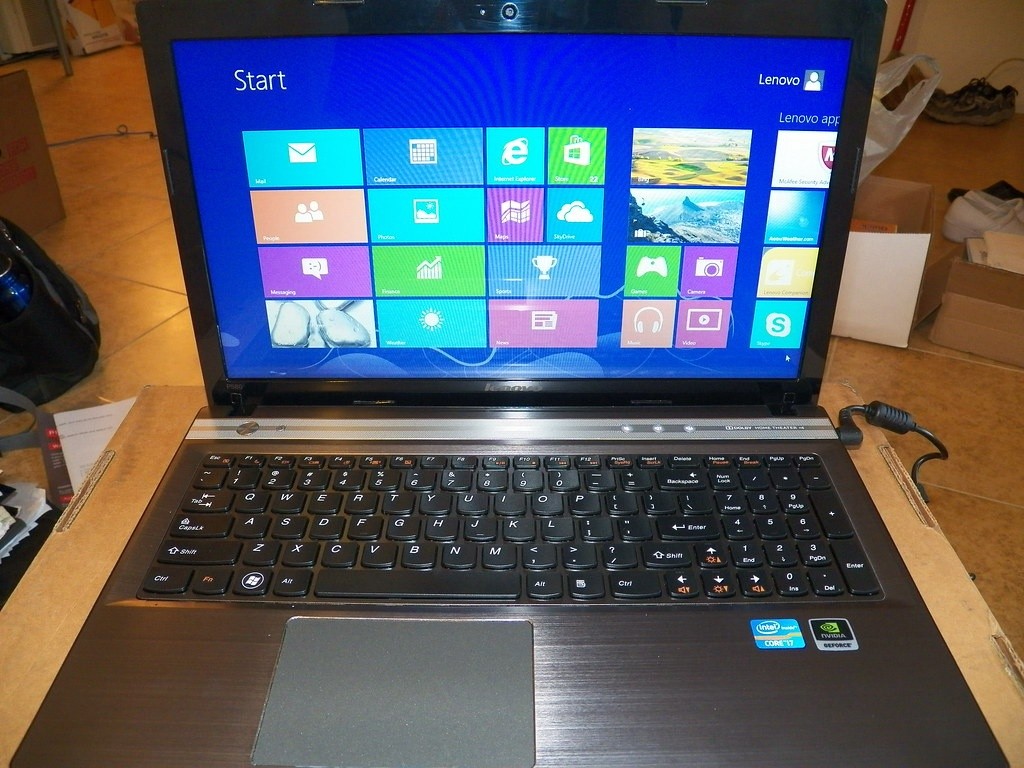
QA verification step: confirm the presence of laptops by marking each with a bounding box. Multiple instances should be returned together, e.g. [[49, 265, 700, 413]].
[[9, 0, 1009, 768]]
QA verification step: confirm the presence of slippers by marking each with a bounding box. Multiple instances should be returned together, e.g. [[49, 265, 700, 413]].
[[947, 180, 1024, 204]]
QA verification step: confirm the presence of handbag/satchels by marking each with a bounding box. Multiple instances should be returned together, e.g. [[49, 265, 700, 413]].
[[0, 215, 101, 413], [857, 54, 944, 188]]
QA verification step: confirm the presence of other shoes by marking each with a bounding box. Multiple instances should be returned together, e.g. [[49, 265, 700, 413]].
[[942, 189, 1024, 243]]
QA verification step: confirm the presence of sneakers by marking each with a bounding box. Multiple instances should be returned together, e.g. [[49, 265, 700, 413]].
[[925, 77, 1018, 126]]
